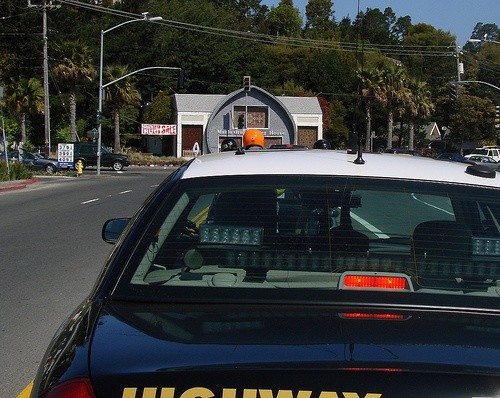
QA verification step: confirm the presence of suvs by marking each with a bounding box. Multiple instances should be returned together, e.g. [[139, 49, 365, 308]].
[[465, 146, 499, 162], [74, 142, 130, 171]]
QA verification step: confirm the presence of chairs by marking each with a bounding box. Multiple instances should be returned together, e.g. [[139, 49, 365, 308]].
[[411, 220, 473, 258], [214, 190, 276, 236]]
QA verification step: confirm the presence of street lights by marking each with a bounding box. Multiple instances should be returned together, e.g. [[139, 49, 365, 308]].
[[95, 11, 164, 175]]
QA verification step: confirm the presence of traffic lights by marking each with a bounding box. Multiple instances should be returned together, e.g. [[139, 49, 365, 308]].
[[238, 114, 245, 128], [177, 69, 186, 90], [243, 76, 251, 92]]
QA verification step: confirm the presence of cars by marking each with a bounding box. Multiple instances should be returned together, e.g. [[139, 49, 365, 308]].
[[0, 150, 61, 174], [31, 142, 499, 398], [385, 147, 424, 158]]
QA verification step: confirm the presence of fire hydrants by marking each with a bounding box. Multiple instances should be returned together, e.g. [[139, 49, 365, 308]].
[[77, 159, 84, 176]]
